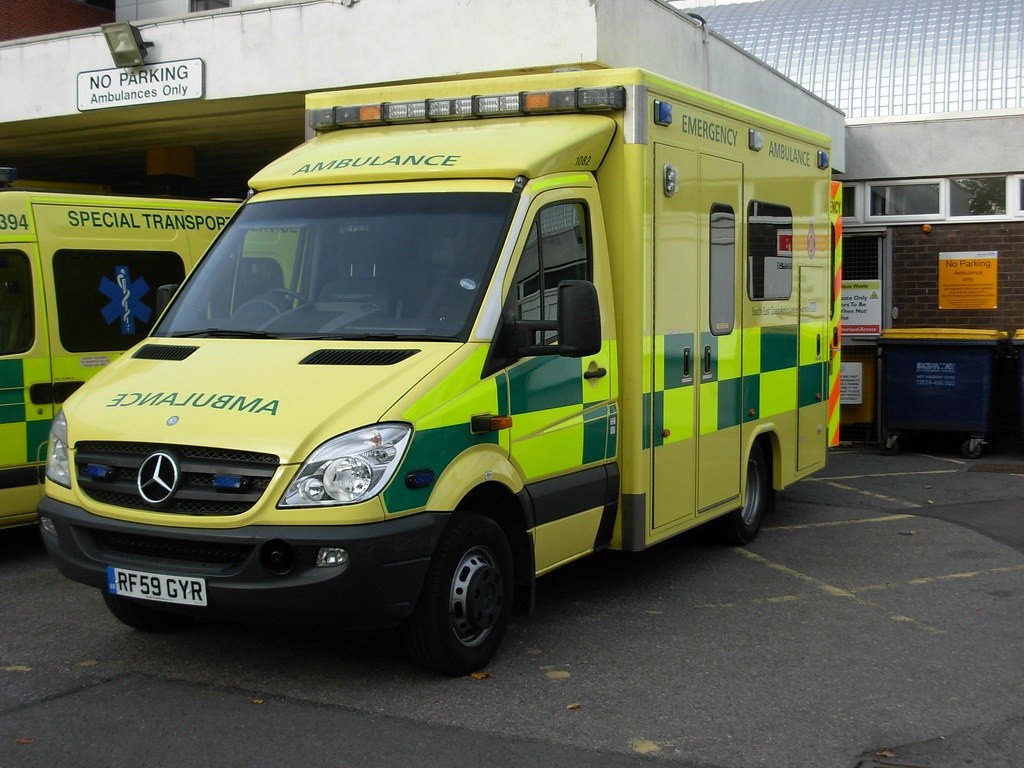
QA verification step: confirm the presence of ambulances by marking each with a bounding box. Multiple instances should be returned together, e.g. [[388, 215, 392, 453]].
[[38, 63, 844, 676], [0, 190, 304, 531]]
